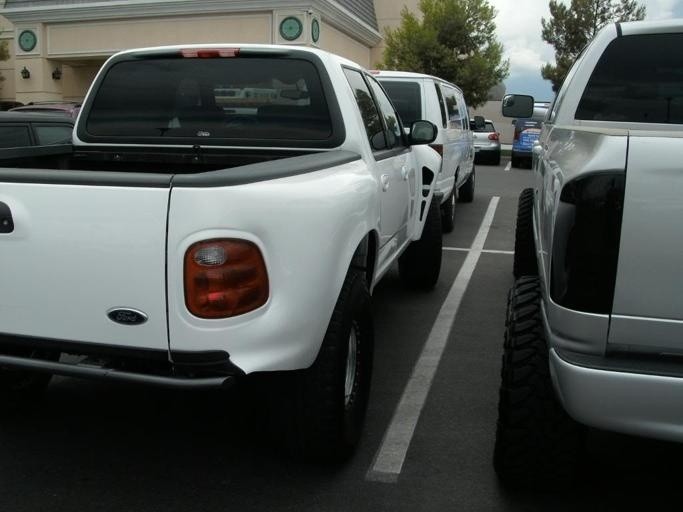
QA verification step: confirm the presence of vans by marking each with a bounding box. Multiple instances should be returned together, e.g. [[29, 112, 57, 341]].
[[296, 71, 486, 232]]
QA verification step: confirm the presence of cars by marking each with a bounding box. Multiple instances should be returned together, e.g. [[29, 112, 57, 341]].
[[510, 118, 548, 165], [466, 120, 502, 165], [0, 99, 84, 168]]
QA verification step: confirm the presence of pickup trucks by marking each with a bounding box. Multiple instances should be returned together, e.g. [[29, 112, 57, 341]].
[[4, 45, 442, 462]]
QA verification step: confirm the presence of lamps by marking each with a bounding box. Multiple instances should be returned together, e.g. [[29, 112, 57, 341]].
[[51, 67, 61, 80], [20, 67, 29, 79]]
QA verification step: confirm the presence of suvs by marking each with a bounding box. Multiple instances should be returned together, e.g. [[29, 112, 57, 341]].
[[501, 17, 682, 443]]
